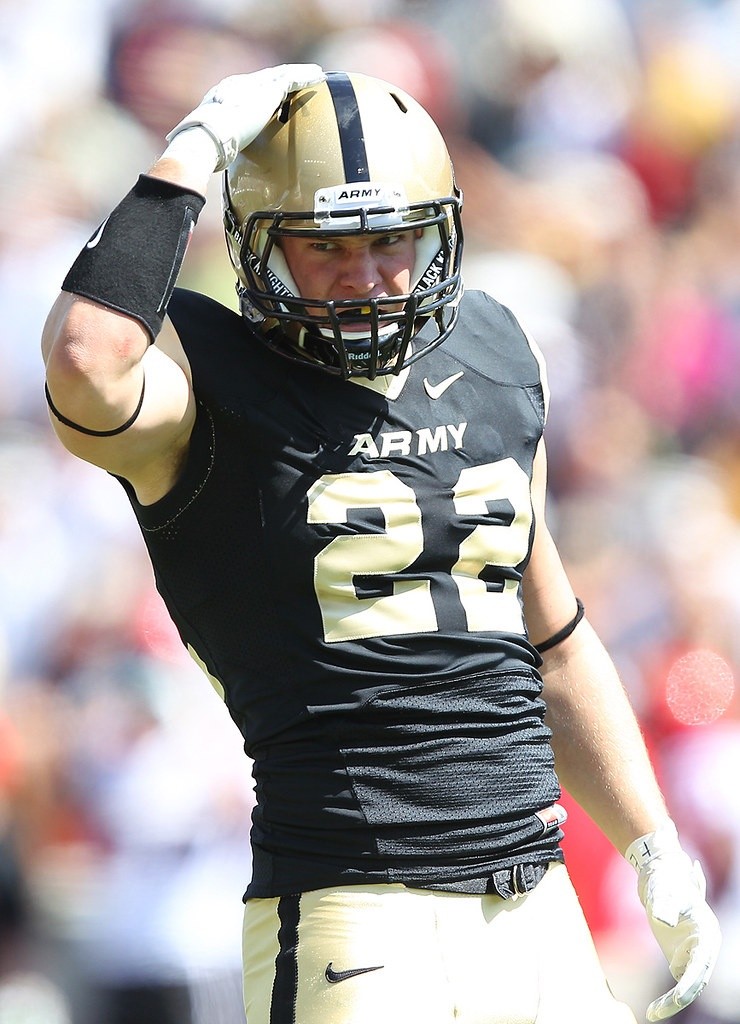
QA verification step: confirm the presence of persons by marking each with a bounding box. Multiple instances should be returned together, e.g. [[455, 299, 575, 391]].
[[40, 62, 720, 1024], [0, 0, 740, 1024]]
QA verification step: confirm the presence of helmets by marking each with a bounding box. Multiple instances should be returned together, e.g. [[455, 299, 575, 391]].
[[222, 72, 453, 371]]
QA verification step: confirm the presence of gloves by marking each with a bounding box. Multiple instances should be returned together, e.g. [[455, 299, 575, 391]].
[[624, 828, 722, 1022], [166, 64, 324, 171]]
